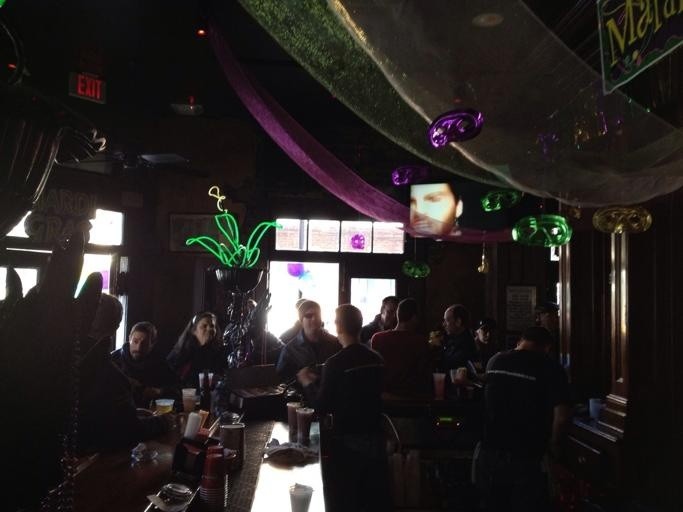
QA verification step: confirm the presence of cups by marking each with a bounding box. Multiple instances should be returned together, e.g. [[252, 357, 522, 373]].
[[450, 369, 467, 398], [199, 444, 227, 509], [182, 387, 195, 410], [218, 422, 245, 462], [286, 401, 314, 441], [198, 372, 212, 389], [154, 398, 175, 415], [288, 484, 312, 511], [432, 372, 447, 398]]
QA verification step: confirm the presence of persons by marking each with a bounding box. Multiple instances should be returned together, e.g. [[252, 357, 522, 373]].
[[410, 183, 464, 238], [77, 313, 227, 512], [246, 296, 572, 512]]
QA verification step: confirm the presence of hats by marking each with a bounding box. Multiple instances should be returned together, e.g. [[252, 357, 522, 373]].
[[294, 299, 317, 322]]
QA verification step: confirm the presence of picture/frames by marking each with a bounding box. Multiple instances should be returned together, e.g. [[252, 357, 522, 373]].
[[165, 210, 240, 255]]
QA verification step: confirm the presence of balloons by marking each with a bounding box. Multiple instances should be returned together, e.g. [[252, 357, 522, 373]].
[[391, 163, 431, 186], [426, 107, 485, 151], [511, 96, 653, 249], [479, 188, 522, 217], [399, 254, 431, 281]]
